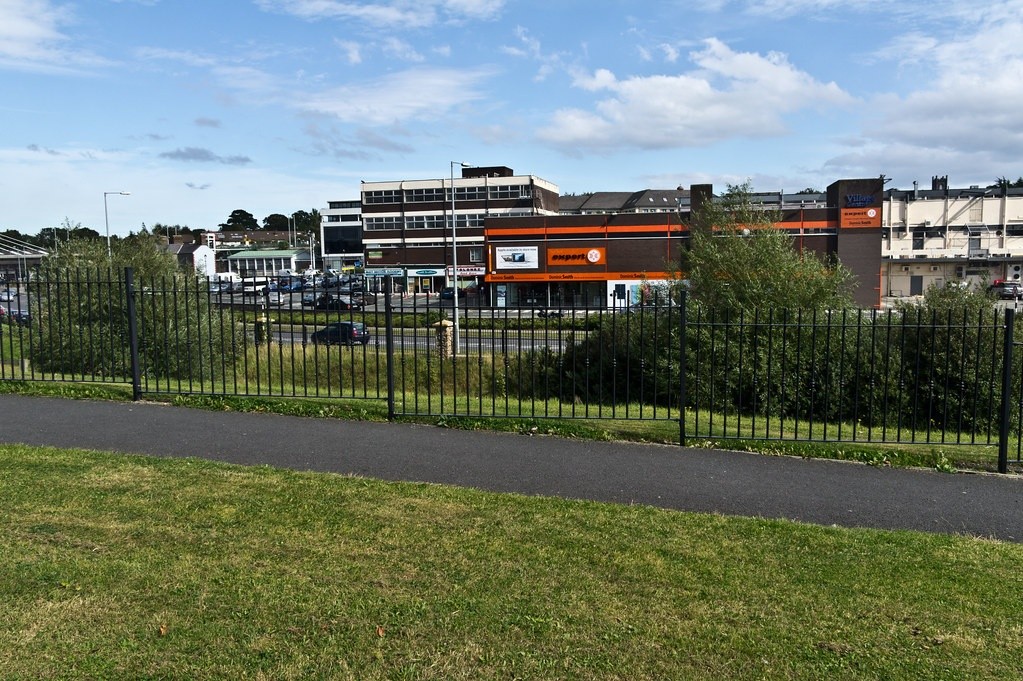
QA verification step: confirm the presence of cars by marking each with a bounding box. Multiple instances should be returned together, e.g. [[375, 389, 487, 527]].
[[0, 288, 34, 328], [208, 267, 377, 313], [441, 287, 467, 300]]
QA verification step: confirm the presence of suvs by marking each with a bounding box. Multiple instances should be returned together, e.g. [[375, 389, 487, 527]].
[[991, 282, 1023, 300], [623, 297, 677, 315], [310, 322, 371, 346]]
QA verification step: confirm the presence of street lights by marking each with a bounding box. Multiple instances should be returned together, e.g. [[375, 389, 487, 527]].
[[104, 192, 131, 260], [450, 160, 470, 355]]
